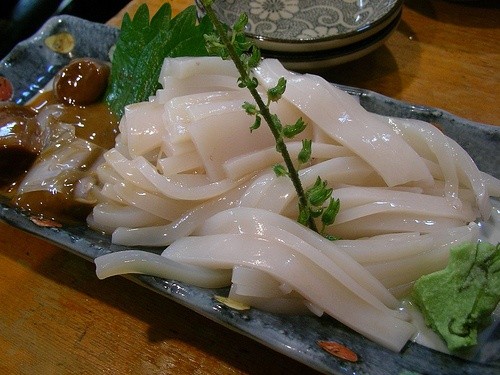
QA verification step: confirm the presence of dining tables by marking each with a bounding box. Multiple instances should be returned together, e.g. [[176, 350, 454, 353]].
[[1, 0, 500, 375]]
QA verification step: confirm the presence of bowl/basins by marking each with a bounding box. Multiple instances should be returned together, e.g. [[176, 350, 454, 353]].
[[195, 0, 405, 72]]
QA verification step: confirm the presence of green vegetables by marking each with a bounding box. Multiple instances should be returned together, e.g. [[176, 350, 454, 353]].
[[105, 2, 232, 119], [198, 0, 341, 242]]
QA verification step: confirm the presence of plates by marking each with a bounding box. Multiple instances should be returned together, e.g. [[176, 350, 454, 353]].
[[0, 17, 500, 375]]
